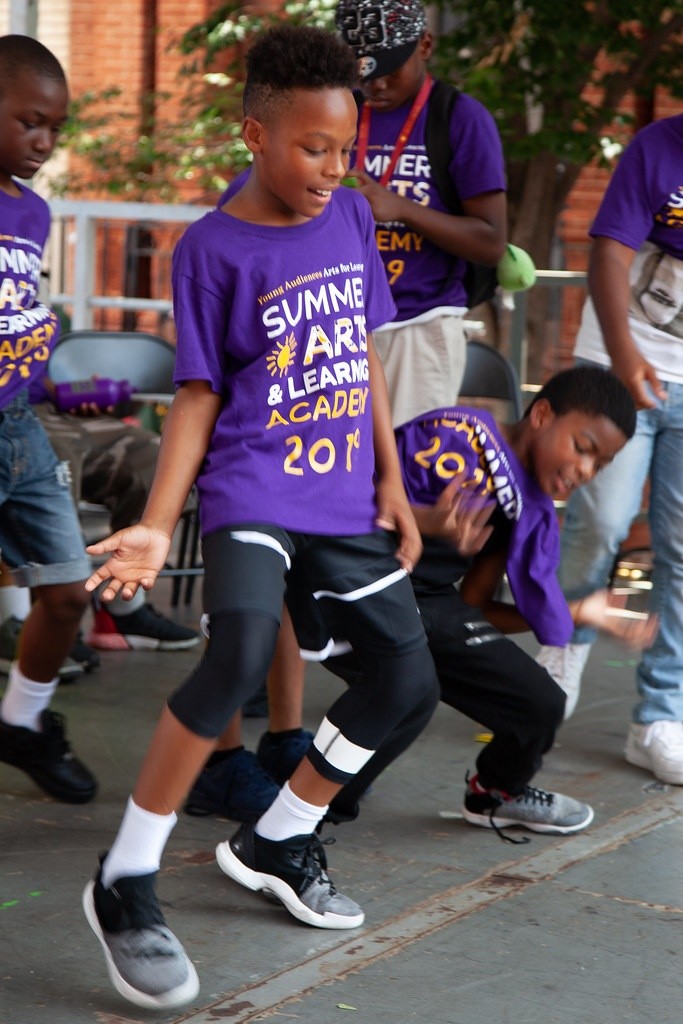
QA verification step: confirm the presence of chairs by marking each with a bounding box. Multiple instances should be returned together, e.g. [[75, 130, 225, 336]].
[[45, 333, 214, 611], [456, 344, 523, 427]]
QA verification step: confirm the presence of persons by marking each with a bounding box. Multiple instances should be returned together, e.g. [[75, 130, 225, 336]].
[[338, 1, 508, 429], [83, 30, 682, 1009], [1, 34, 98, 806], [0, 367, 201, 685]]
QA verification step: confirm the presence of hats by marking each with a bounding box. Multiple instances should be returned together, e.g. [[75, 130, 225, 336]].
[[335, 0, 426, 80]]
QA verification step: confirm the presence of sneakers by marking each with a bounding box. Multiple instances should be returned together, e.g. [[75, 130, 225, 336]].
[[624, 719, 682, 785], [215, 811, 366, 929], [1, 708, 96, 803], [462, 769, 594, 844], [89, 602, 201, 650], [537, 641, 590, 720], [258, 730, 371, 798], [83, 849, 199, 1010], [184, 749, 282, 822], [1, 616, 85, 679]]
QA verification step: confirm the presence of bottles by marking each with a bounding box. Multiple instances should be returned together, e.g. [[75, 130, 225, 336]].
[[493, 241, 537, 294], [52, 381, 130, 415]]
